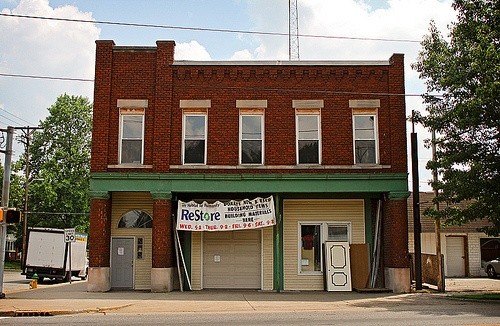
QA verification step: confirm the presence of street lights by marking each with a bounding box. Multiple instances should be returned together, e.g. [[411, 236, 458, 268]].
[[22, 178, 45, 269]]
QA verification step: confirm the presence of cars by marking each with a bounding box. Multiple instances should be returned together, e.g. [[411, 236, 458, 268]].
[[484, 257, 500, 278]]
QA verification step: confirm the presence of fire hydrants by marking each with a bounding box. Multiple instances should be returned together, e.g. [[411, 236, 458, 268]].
[[28, 273, 39, 289]]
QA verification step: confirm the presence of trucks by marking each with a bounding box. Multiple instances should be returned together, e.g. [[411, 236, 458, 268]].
[[21, 226, 89, 281]]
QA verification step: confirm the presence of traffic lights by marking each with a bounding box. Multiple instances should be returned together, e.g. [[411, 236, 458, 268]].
[[6, 211, 22, 224]]
